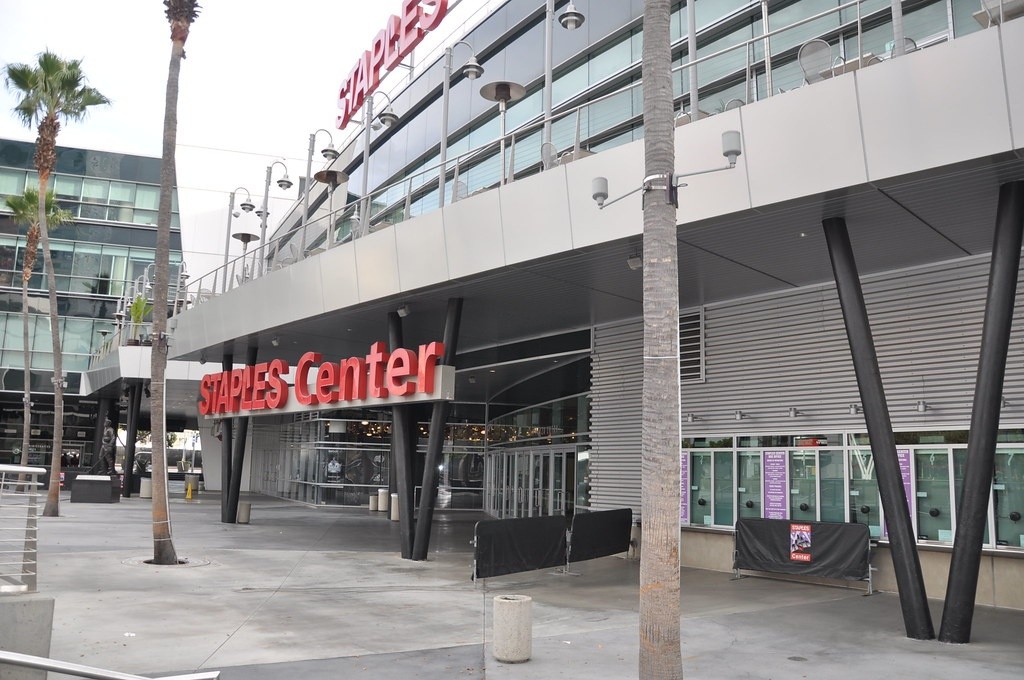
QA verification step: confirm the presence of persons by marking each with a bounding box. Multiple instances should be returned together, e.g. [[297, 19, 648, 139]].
[[70, 454, 79, 467], [61, 453, 67, 467], [66, 453, 72, 467], [97, 410, 117, 475]]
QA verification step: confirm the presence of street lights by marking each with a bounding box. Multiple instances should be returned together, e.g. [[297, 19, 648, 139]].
[[298, 129, 340, 262], [544, 0, 585, 169], [223, 187, 255, 293], [258, 161, 294, 278], [360, 91, 399, 233], [439, 41, 484, 205], [99, 260, 190, 357]]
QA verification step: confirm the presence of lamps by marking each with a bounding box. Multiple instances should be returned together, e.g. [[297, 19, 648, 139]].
[[686, 399, 933, 424], [272, 336, 281, 347], [397, 305, 411, 318], [627, 252, 643, 271]]
